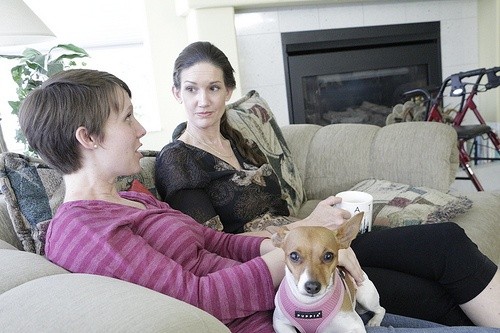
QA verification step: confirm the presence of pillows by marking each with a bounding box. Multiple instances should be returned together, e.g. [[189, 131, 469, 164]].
[[223, 88, 308, 217], [0, 150, 168, 255]]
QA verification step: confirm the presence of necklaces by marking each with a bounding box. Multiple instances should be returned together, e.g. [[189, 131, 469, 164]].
[[185, 129, 233, 158]]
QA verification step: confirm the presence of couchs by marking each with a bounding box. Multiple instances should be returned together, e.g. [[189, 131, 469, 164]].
[[0, 120, 459, 333]]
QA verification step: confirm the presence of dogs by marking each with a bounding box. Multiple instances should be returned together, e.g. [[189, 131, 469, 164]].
[[265, 211, 386, 332]]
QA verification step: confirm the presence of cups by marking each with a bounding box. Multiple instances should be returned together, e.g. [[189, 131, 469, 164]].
[[336, 191, 374, 234]]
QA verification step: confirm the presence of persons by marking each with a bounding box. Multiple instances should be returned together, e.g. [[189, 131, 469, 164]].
[[153, 41, 500, 328], [19, 69, 500, 333]]
